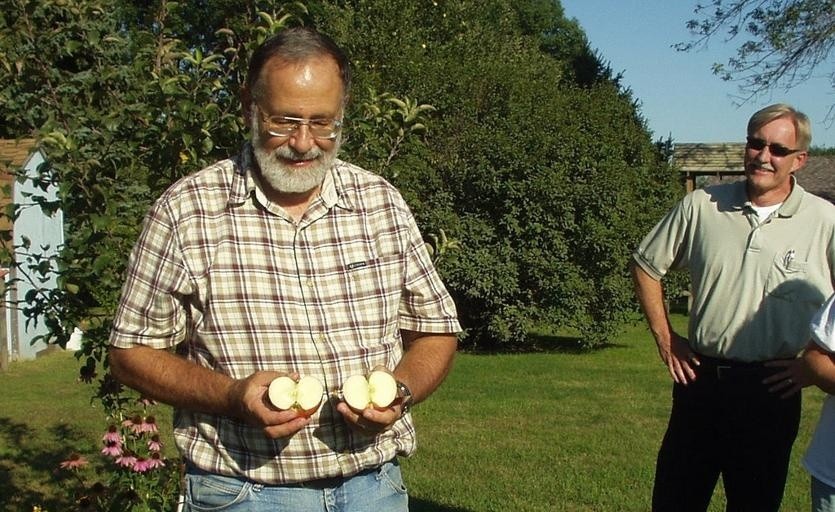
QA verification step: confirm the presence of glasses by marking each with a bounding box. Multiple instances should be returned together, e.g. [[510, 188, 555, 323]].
[[744, 134, 800, 158], [244, 83, 346, 139]]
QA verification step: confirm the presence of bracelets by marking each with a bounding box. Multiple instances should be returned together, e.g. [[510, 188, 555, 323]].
[[395, 381, 414, 413]]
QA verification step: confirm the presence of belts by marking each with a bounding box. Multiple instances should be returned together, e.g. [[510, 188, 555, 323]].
[[687, 353, 770, 383]]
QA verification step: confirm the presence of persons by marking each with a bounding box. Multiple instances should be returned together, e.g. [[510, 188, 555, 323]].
[[800, 290, 835, 511], [105, 29, 462, 512], [632, 104, 835, 510]]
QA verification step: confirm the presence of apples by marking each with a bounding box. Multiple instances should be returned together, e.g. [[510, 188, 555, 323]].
[[269, 375, 323, 419], [342, 370, 398, 414]]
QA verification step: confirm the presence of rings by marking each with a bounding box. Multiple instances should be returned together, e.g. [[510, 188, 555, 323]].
[[787, 377, 793, 382]]
